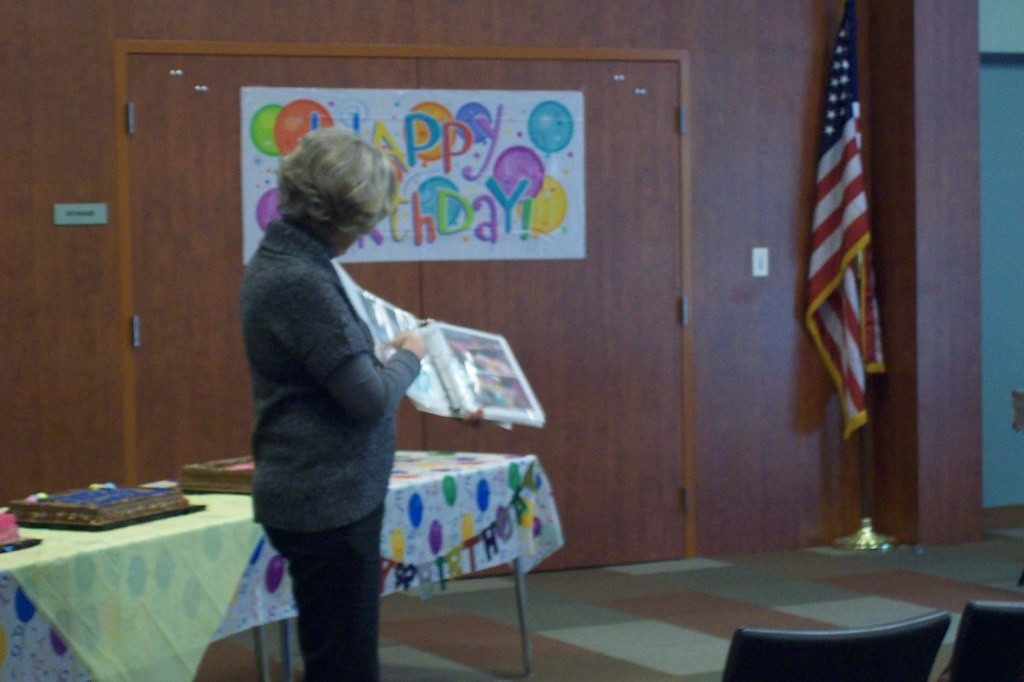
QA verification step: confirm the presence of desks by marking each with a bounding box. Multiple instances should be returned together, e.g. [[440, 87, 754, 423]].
[[0, 450, 564, 682]]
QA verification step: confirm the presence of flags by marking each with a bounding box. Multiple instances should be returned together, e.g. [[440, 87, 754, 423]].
[[805, 20, 888, 440]]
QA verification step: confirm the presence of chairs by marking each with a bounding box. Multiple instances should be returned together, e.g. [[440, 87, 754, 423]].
[[717, 599, 1024, 682]]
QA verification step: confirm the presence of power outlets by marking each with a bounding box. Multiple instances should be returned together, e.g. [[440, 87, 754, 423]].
[[752, 247, 769, 277]]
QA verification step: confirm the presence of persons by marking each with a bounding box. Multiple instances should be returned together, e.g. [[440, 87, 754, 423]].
[[240, 128, 430, 682]]
